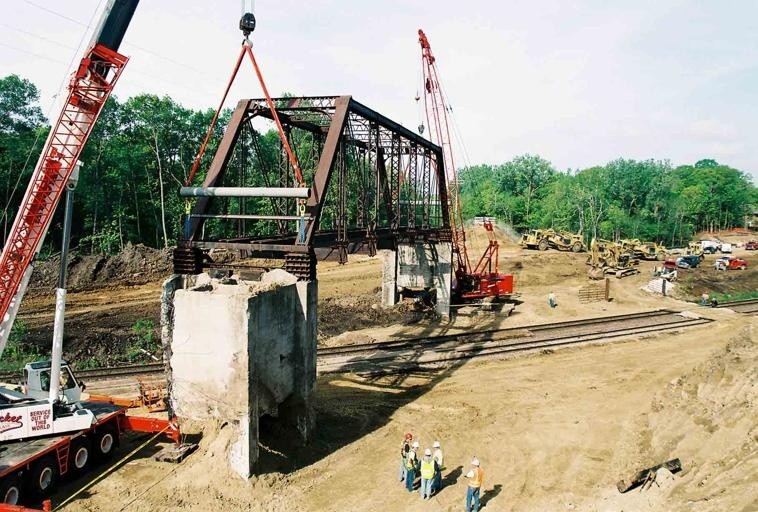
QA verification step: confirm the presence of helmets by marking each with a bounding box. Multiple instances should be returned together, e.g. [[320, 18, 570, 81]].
[[411, 442, 419, 448], [471, 459, 480, 466], [405, 433, 412, 439], [712, 298, 715, 301], [424, 448, 431, 455], [703, 290, 709, 294], [433, 441, 440, 448]]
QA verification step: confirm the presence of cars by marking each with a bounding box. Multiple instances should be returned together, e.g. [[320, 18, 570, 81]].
[[697, 237, 732, 254], [715, 256, 748, 271], [745, 240, 758, 250]]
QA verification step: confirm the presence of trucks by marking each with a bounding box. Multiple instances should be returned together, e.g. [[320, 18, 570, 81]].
[[664, 256, 702, 269]]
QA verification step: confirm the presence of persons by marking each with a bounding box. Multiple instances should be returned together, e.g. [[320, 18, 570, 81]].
[[546, 289, 557, 310], [466, 457, 484, 512], [701, 289, 718, 308], [399, 432, 442, 500]]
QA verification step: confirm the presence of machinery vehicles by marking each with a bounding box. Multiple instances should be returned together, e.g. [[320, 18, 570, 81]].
[[679, 243, 705, 262], [410, 28, 513, 310], [633, 239, 671, 261], [518, 229, 586, 252], [1, 0, 313, 511], [586, 238, 639, 280]]
